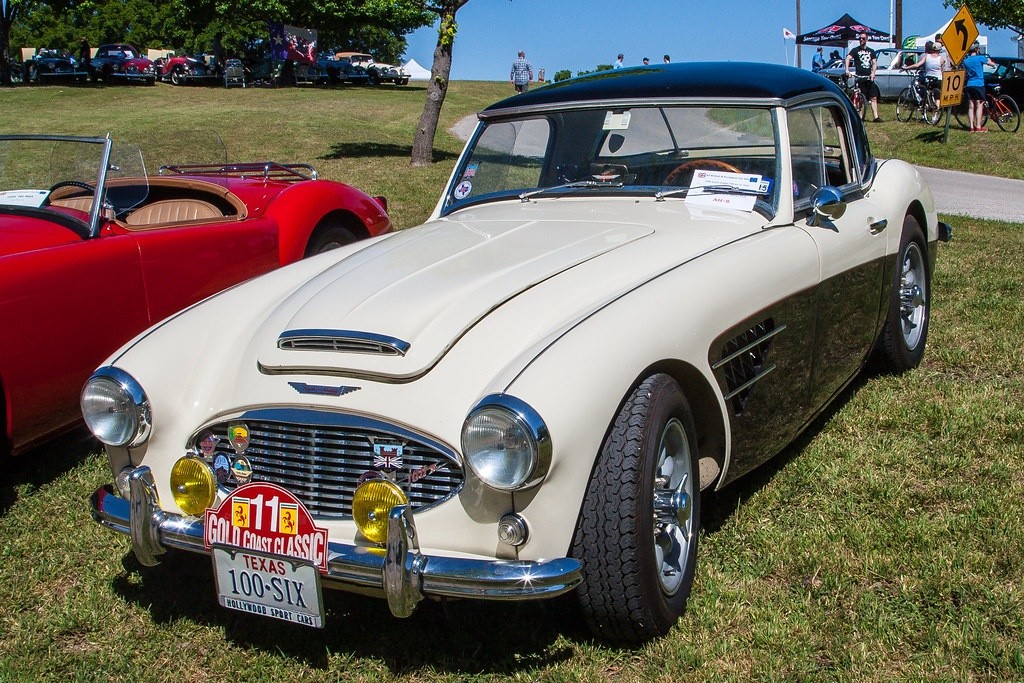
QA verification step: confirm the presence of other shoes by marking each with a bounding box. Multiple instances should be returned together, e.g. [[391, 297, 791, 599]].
[[975, 127, 988, 133], [919, 118, 928, 124], [930, 117, 938, 123], [970, 126, 975, 132], [873, 117, 883, 123]]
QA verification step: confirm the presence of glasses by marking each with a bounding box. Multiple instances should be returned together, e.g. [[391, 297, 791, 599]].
[[859, 37, 867, 40]]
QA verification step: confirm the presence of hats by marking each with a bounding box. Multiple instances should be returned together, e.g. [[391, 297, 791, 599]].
[[932, 42, 942, 50], [925, 41, 933, 47], [969, 41, 979, 49]]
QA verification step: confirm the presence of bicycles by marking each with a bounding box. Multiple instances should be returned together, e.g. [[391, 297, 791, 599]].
[[953, 82, 1021, 133], [838, 72, 872, 121], [895, 68, 945, 126]]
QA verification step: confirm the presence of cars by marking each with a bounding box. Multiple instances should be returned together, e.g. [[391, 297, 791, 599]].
[[956, 56, 1024, 112], [0, 133, 394, 483], [817, 48, 944, 99], [27, 52, 88, 86], [86, 44, 157, 85], [244, 52, 409, 86], [79, 62, 950, 651], [154, 50, 221, 85]]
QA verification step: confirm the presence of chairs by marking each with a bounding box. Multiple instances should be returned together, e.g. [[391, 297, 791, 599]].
[[51, 196, 116, 219], [223, 59, 246, 88], [123, 198, 224, 225]]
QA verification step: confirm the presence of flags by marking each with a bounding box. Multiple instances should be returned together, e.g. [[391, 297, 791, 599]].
[[266, 18, 316, 65]]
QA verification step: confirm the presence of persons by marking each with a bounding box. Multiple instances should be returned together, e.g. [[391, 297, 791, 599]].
[[213, 32, 225, 75], [511, 50, 533, 94], [222, 49, 236, 65], [643, 58, 649, 65], [80, 38, 91, 60], [812, 47, 822, 70], [36, 48, 48, 61], [962, 40, 996, 132], [830, 50, 842, 60], [845, 32, 883, 122], [905, 53, 914, 66], [664, 55, 670, 63], [614, 53, 624, 69], [902, 34, 952, 123]]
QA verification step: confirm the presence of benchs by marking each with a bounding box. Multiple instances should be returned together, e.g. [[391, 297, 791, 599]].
[[627, 157, 846, 204]]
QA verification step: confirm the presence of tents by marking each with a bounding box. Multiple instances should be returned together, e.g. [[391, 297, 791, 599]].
[[915, 19, 987, 63], [794, 13, 895, 67]]
